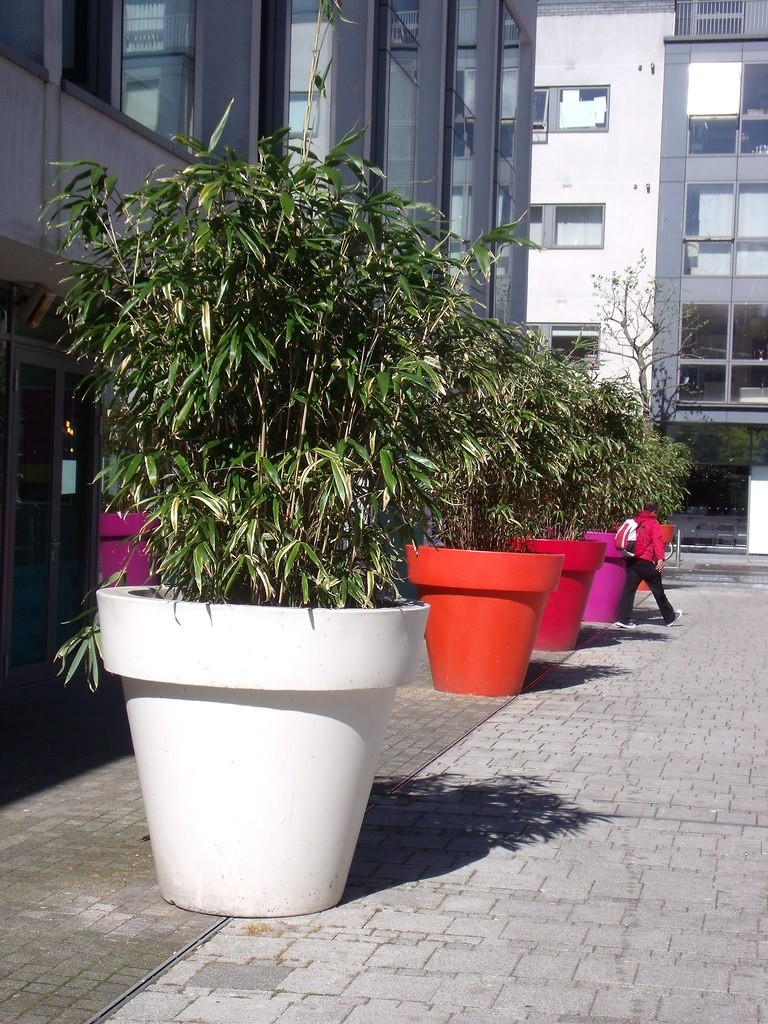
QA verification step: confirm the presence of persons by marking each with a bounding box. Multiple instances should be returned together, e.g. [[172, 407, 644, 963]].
[[616, 500, 683, 628], [752, 346, 768, 387]]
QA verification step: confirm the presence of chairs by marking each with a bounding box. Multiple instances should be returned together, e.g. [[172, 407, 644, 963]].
[[695, 522, 716, 546], [716, 524, 735, 546]]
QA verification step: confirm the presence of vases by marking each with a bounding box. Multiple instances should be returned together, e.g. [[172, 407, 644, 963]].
[[97, 512, 162, 586]]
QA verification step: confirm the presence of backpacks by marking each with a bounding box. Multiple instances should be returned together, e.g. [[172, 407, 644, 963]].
[[614, 514, 649, 558]]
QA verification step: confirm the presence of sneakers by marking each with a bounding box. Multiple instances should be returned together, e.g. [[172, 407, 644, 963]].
[[616, 620, 637, 629], [666, 610, 682, 627]]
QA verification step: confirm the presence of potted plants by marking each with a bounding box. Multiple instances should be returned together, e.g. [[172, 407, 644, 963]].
[[33, 1, 546, 919], [505, 362, 614, 651], [548, 371, 667, 624], [612, 436, 693, 591], [404, 326, 606, 698]]
